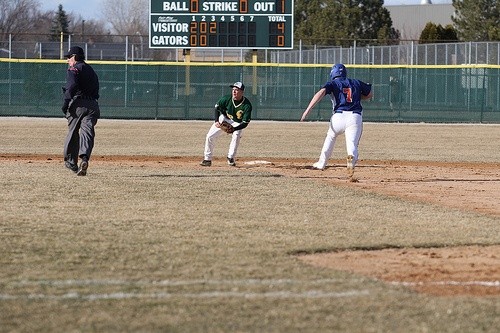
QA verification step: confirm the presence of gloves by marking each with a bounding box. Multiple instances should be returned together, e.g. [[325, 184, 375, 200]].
[[62, 99, 71, 114]]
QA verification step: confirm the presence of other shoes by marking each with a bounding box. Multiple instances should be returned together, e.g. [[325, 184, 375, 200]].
[[227, 156, 236, 166], [312, 162, 325, 170], [199, 160, 211, 167], [347, 155, 355, 173]]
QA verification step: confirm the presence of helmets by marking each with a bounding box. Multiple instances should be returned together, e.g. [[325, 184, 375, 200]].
[[330, 63, 346, 80]]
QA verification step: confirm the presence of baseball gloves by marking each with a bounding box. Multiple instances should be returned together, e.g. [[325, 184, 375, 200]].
[[219, 120, 235, 135]]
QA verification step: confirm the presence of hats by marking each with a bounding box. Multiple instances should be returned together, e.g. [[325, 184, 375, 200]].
[[64, 46, 85, 57], [230, 82, 245, 90]]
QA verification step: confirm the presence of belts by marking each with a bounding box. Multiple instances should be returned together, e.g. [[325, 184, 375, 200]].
[[335, 110, 361, 115]]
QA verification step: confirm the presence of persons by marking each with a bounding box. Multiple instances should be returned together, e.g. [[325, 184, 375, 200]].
[[62, 45, 100, 176], [390, 76, 406, 113], [200, 82, 253, 166], [300, 63, 373, 177]]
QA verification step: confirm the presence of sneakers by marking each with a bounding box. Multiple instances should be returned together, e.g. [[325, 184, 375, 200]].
[[76, 160, 88, 176], [64, 161, 78, 171]]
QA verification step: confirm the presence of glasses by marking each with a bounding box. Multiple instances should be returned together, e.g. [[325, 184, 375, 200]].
[[68, 55, 74, 59]]
